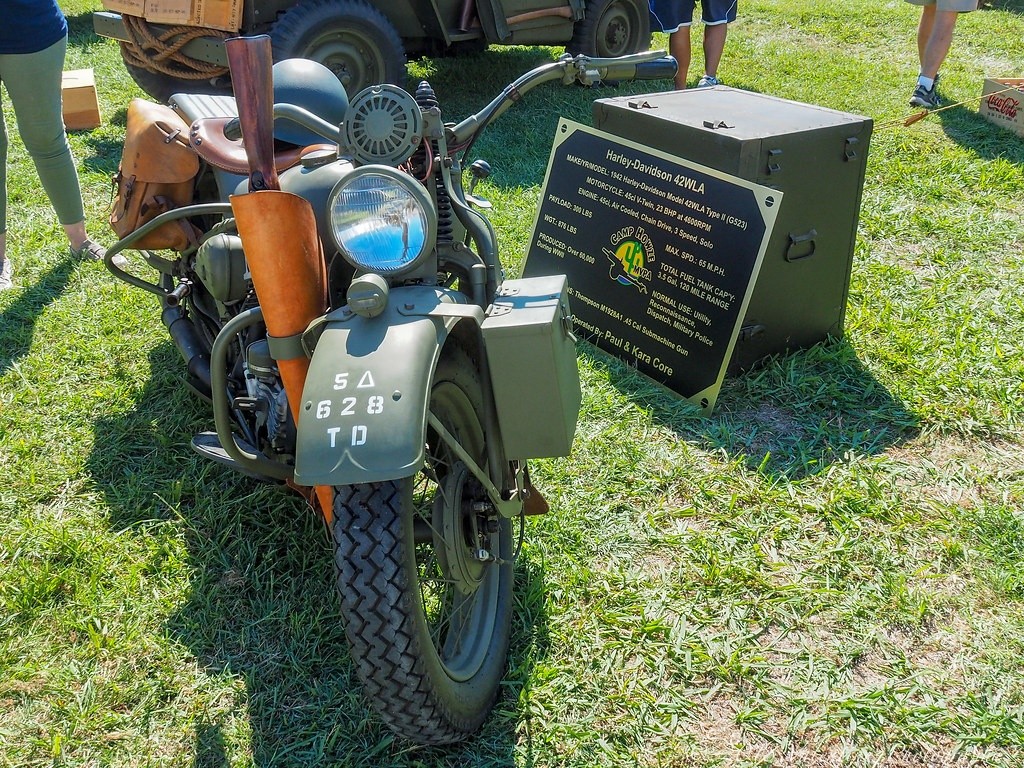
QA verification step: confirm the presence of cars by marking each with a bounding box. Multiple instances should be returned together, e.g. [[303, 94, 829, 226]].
[[89, 0, 656, 110]]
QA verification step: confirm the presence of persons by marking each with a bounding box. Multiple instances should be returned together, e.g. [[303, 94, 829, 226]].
[[0, 0, 126, 293], [648, 0, 737, 90], [903, 0, 979, 108]]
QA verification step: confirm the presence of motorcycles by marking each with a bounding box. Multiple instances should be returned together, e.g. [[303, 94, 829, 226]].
[[102, 51, 680, 750]]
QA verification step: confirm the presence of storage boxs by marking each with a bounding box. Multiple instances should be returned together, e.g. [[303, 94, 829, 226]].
[[483, 275, 582, 460], [590, 83, 875, 378], [978, 77, 1024, 138], [101, 0, 144, 18], [144, 0, 244, 33], [61, 68, 103, 130]]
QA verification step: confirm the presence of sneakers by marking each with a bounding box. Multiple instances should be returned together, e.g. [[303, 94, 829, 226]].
[[0, 258, 12, 290], [909, 72, 941, 108], [70, 240, 126, 267]]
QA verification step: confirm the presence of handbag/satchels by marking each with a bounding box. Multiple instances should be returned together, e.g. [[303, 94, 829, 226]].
[[106, 98, 198, 251]]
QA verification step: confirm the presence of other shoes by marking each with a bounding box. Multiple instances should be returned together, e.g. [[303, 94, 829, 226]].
[[697, 75, 717, 88]]
